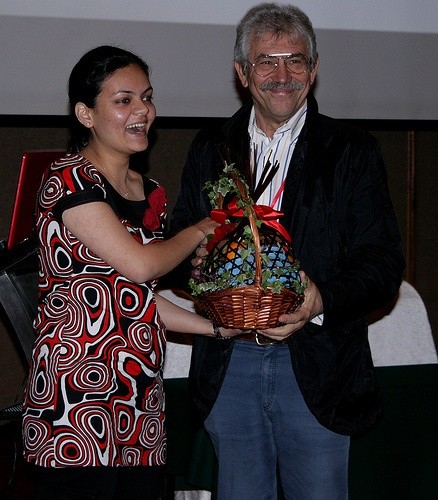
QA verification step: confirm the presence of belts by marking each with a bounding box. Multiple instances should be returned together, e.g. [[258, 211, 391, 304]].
[[236, 332, 289, 346]]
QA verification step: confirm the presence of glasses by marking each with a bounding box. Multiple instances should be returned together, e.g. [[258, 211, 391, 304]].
[[247, 53, 310, 77]]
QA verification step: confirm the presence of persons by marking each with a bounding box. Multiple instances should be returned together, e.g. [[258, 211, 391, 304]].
[[167, 3, 405, 500], [21, 46, 254, 500]]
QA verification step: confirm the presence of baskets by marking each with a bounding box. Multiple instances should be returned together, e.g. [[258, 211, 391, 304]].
[[191, 166, 304, 330]]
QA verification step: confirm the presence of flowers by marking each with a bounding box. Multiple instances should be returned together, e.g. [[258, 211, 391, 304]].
[[142, 187, 168, 231]]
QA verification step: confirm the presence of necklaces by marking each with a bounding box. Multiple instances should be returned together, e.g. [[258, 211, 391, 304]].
[[93, 155, 130, 198]]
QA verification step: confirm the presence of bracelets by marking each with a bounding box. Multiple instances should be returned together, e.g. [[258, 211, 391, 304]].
[[212, 322, 230, 340]]
